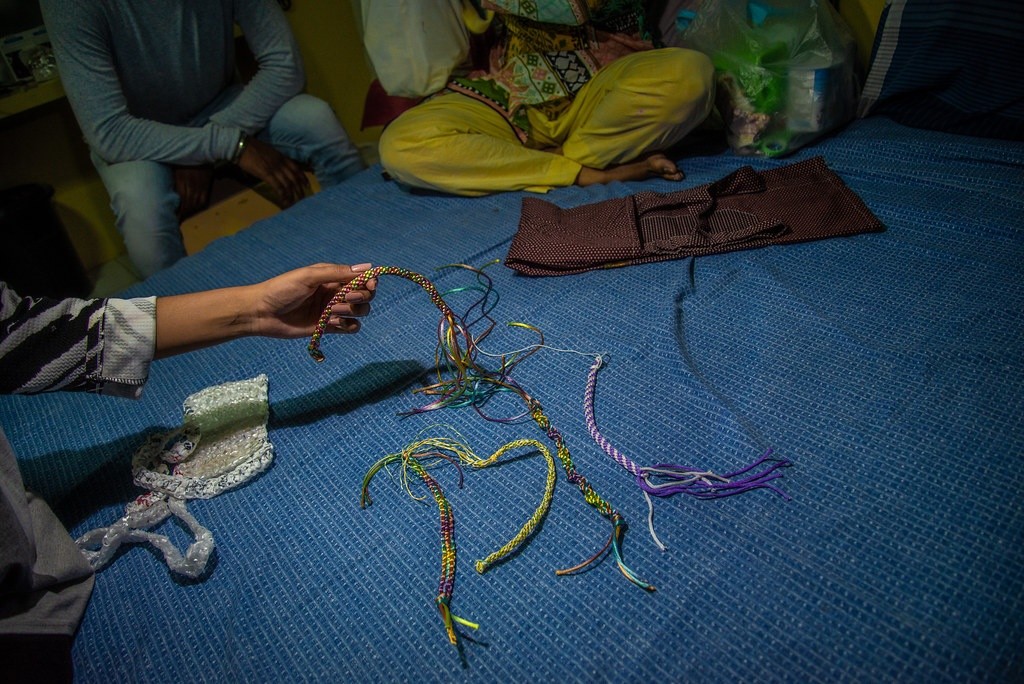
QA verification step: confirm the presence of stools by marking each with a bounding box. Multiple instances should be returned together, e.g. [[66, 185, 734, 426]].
[[177, 158, 324, 258]]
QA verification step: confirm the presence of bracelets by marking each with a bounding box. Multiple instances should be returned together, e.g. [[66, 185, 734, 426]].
[[232, 130, 247, 170]]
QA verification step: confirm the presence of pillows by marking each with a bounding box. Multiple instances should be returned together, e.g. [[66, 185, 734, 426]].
[[856, 1, 1024, 142]]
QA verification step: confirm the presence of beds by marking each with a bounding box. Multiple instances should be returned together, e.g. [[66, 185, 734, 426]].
[[1, 114, 1024, 682]]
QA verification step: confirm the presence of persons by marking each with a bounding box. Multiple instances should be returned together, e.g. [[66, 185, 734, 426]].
[[39, 0, 367, 280], [0, 263, 378, 684], [361, 0, 717, 199]]
